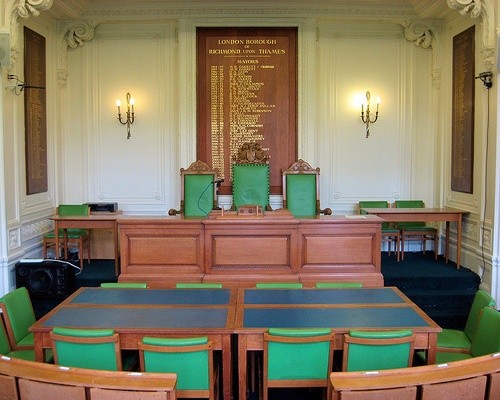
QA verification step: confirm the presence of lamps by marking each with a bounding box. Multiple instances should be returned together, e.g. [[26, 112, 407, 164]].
[[116, 92, 135, 140], [361, 90, 380, 138]]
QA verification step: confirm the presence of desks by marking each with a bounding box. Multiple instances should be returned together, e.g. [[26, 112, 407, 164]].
[[49, 209, 122, 278], [360, 207, 470, 269], [235, 287, 443, 400], [117, 214, 386, 286], [27, 287, 238, 400]]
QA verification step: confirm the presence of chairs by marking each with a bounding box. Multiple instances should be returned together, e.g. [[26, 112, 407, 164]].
[[282, 158, 333, 215], [229, 142, 274, 211], [168, 159, 221, 217], [0, 283, 500, 400], [44, 204, 91, 269], [359, 200, 439, 263]]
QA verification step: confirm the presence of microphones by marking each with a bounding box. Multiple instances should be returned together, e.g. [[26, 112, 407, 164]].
[[211, 179, 224, 184]]
